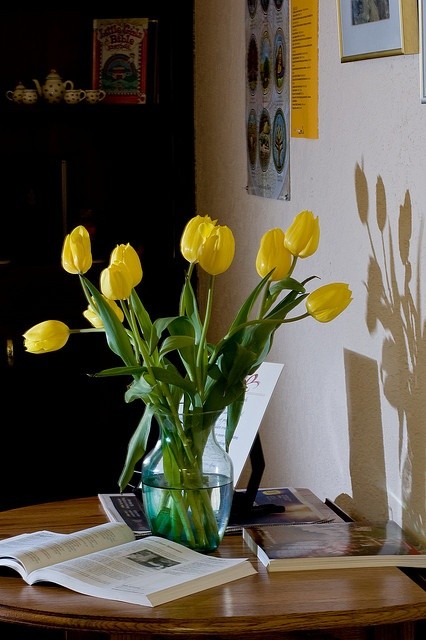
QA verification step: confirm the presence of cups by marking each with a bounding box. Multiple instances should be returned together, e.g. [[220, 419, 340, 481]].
[[84, 89, 108, 104], [15, 89, 38, 104], [62, 88, 87, 104]]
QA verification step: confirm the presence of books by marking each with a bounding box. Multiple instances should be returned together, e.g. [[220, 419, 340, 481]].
[[0, 522, 259, 607], [240, 518, 426, 570]]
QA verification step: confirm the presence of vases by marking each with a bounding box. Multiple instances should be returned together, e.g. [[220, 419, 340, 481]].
[[141, 406, 234, 552]]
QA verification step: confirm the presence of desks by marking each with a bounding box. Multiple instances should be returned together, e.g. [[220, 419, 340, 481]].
[[0, 489, 426, 640]]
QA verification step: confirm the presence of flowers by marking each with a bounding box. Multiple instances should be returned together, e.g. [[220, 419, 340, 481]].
[[22, 210, 354, 549]]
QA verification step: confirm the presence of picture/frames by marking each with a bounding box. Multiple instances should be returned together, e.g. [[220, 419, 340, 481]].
[[334, 0, 419, 64]]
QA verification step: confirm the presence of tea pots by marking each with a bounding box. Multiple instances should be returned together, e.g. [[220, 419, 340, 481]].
[[33, 68, 73, 103], [6, 82, 29, 103]]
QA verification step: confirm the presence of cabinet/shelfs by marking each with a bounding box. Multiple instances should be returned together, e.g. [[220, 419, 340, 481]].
[[0, 0, 194, 513]]
